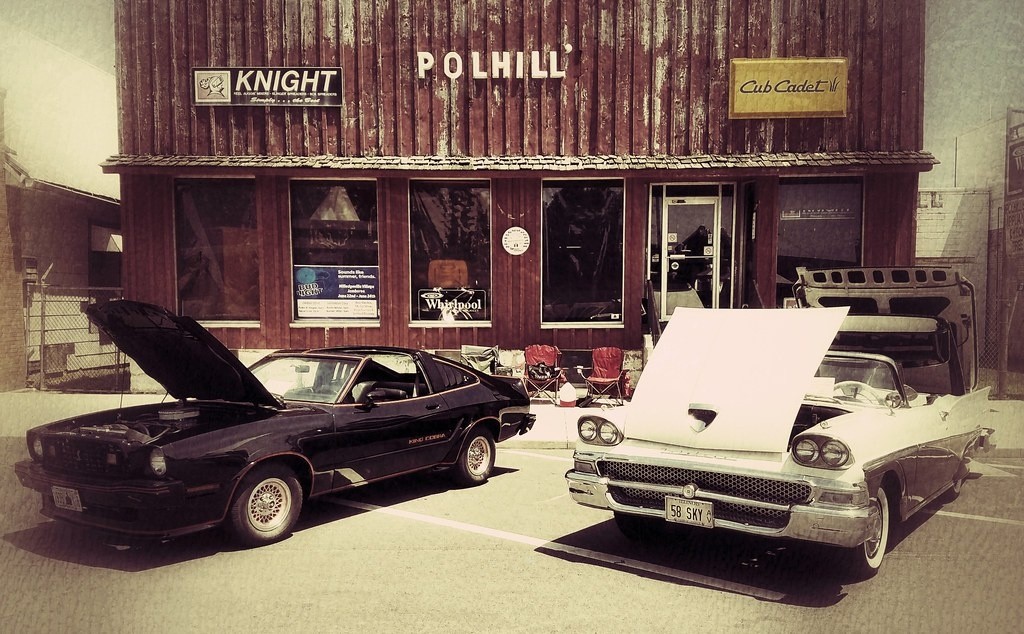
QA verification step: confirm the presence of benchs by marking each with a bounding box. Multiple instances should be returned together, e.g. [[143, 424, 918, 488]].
[[908, 392, 931, 407], [378, 381, 428, 403]]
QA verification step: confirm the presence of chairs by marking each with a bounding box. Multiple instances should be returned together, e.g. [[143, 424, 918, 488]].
[[317, 385, 341, 395], [460, 344, 499, 377], [857, 387, 894, 406], [522, 344, 561, 406], [584, 346, 624, 408], [366, 388, 408, 404]]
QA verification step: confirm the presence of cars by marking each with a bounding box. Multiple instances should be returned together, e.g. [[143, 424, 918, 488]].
[[564, 262, 992, 580], [544, 290, 621, 321], [14, 300, 537, 547]]
